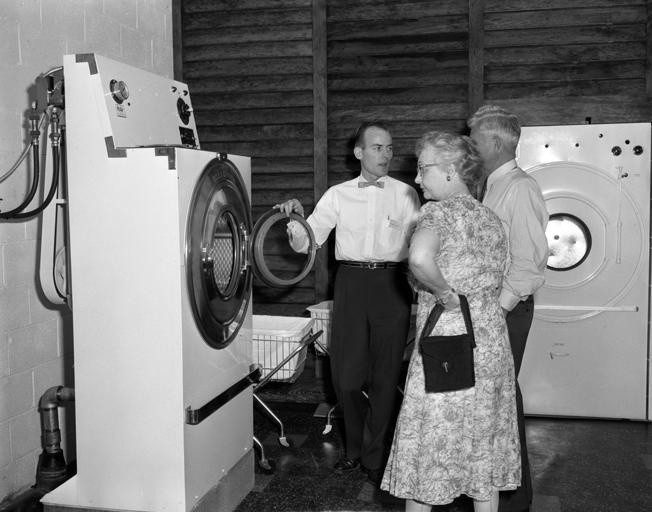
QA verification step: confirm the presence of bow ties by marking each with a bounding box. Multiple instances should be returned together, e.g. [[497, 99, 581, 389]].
[[358, 182, 384, 189]]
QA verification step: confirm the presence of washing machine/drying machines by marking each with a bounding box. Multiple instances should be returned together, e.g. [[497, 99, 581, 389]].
[[32, 54, 317, 512], [511, 122, 650, 421]]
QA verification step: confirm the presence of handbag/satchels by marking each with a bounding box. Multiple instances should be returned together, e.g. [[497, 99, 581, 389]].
[[419, 295, 476, 393]]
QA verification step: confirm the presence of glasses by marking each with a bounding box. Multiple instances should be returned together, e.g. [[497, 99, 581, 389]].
[[417, 162, 440, 173]]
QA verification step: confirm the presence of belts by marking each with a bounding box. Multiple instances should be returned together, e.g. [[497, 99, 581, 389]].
[[341, 260, 403, 270]]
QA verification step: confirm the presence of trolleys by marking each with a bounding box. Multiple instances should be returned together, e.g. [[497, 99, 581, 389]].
[[310, 313, 416, 442], [252, 330, 326, 476]]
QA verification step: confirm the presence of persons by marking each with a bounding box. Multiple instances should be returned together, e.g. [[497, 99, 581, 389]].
[[400, 129, 511, 512], [464, 102, 550, 512], [272, 121, 422, 487]]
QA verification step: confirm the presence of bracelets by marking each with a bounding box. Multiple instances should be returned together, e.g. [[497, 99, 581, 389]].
[[434, 287, 456, 305]]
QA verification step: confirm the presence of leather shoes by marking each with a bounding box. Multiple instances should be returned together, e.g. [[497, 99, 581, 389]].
[[333, 459, 361, 474]]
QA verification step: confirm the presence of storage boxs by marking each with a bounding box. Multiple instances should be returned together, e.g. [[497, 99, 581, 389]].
[[252, 296, 334, 383]]
